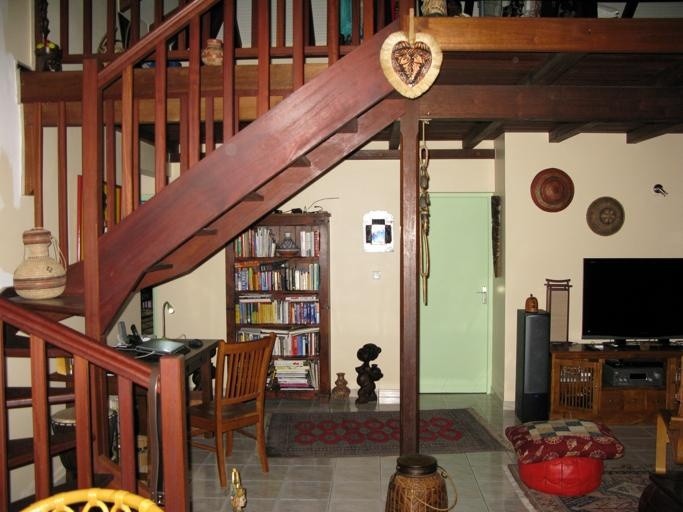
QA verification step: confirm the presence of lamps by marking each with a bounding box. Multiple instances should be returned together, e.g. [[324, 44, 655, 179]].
[[162, 301, 175, 338]]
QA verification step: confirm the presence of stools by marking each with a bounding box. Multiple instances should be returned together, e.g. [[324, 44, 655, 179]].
[[518, 455, 603, 497]]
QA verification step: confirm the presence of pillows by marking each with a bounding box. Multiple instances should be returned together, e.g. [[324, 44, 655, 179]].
[[505, 417, 625, 464]]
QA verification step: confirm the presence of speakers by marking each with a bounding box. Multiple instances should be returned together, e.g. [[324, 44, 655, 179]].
[[515, 309, 550, 423]]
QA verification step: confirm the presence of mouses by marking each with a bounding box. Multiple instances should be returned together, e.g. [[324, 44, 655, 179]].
[[189, 339, 203, 347]]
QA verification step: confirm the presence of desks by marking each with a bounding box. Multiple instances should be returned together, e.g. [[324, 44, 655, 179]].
[[66, 338, 219, 482]]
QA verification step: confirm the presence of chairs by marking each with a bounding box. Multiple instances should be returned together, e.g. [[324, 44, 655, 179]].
[[21, 487, 166, 512], [189, 332, 276, 487], [655, 355, 683, 475]]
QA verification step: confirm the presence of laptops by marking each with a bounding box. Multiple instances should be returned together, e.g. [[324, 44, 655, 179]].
[[136, 340, 185, 355]]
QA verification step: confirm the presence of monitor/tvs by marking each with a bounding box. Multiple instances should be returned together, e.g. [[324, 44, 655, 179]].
[[582, 258, 683, 351]]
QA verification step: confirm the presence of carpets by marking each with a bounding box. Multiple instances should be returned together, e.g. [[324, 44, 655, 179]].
[[264, 407, 512, 458], [500, 460, 655, 511]]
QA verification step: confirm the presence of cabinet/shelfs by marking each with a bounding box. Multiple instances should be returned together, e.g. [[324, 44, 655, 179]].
[[549, 341, 683, 425], [225, 216, 331, 404]]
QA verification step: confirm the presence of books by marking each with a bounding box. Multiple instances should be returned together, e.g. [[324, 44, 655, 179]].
[[230, 229, 320, 392]]
